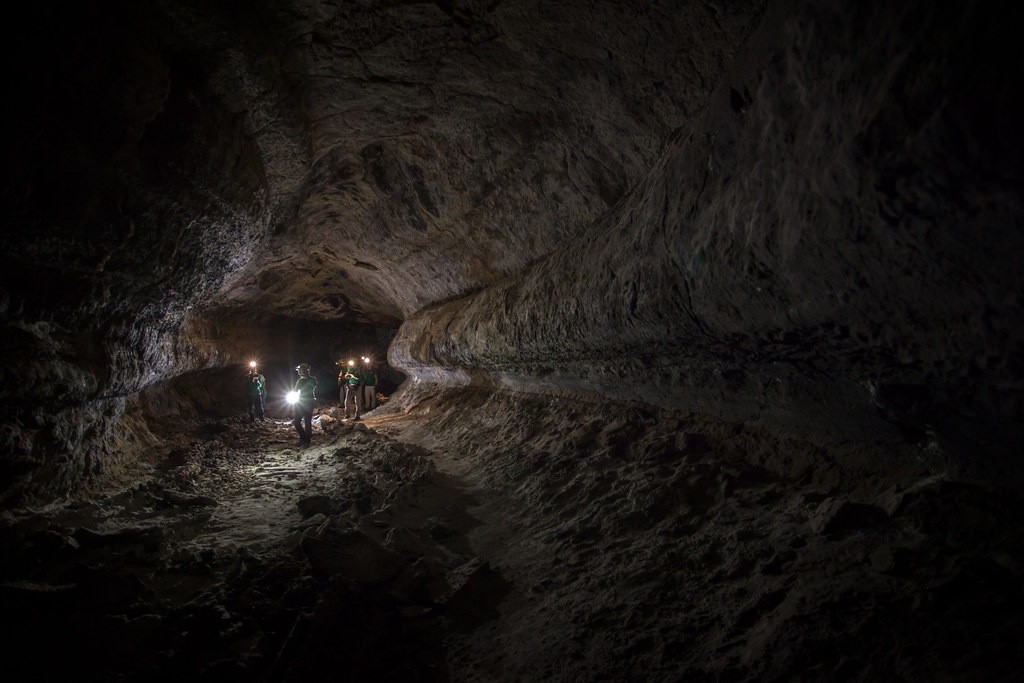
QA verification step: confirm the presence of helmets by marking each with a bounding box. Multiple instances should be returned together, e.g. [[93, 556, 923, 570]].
[[295, 363, 311, 370]]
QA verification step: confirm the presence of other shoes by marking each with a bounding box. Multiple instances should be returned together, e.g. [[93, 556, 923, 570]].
[[296, 440, 310, 449], [352, 417, 360, 420], [345, 414, 350, 419]]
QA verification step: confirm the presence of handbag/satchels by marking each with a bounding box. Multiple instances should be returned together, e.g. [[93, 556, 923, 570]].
[[348, 385, 357, 391]]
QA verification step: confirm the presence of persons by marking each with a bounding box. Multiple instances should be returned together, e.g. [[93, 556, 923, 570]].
[[246, 368, 266, 423], [335, 357, 379, 421], [293, 363, 318, 449]]
